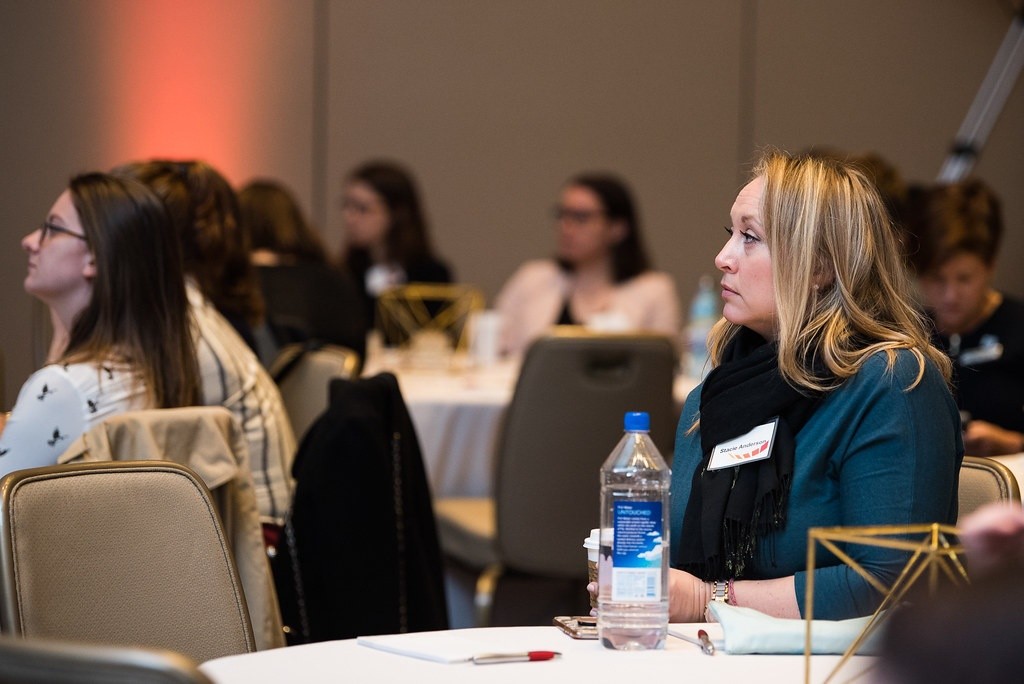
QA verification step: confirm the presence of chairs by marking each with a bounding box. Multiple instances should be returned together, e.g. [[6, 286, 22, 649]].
[[1, 326, 685, 684], [957, 455, 1020, 517]]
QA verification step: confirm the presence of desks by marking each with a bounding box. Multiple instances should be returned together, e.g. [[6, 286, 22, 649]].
[[199, 622, 876, 684], [364, 359, 709, 629]]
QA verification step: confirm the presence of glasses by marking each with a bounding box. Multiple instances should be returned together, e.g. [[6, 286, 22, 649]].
[[38, 219, 90, 246]]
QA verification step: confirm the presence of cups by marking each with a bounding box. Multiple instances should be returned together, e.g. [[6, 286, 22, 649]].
[[466, 312, 500, 375], [583, 528, 669, 618]]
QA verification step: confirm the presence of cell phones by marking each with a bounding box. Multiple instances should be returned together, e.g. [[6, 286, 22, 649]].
[[553, 615, 600, 640]]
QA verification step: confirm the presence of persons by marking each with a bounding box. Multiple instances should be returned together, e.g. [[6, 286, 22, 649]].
[[341, 158, 454, 332], [495, 170, 682, 363], [912, 181, 1024, 456], [238, 182, 358, 350], [0, 159, 297, 529], [587, 159, 966, 622]]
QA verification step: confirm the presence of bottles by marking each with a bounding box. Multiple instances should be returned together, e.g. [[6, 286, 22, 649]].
[[600, 413, 672, 652], [684, 277, 717, 367]]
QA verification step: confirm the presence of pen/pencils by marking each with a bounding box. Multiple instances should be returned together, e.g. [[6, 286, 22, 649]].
[[473, 651, 556, 665]]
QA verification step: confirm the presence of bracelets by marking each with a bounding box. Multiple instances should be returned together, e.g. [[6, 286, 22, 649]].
[[729, 581, 736, 605]]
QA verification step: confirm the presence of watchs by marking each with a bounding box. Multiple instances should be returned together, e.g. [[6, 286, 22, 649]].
[[704, 581, 729, 622]]
